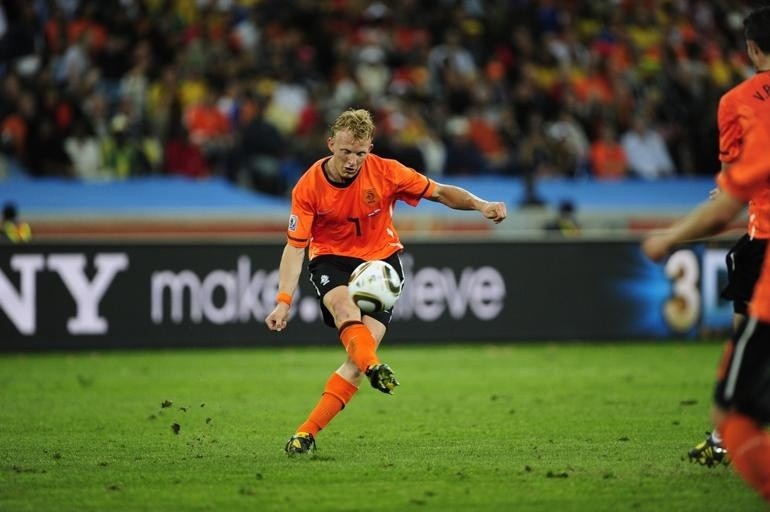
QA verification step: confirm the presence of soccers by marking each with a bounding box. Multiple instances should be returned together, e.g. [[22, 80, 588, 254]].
[[348, 260, 401, 314]]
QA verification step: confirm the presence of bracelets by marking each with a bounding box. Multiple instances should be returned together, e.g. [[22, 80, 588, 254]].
[[275, 292, 293, 308]]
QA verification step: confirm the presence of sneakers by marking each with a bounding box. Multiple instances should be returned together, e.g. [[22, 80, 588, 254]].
[[285, 433, 316, 456], [366, 363, 400, 395], [687, 434, 730, 466]]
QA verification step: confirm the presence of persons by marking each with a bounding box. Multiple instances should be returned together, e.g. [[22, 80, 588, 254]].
[[264, 108, 507, 457], [642, 5, 770, 502], [0, 0, 769, 244]]
